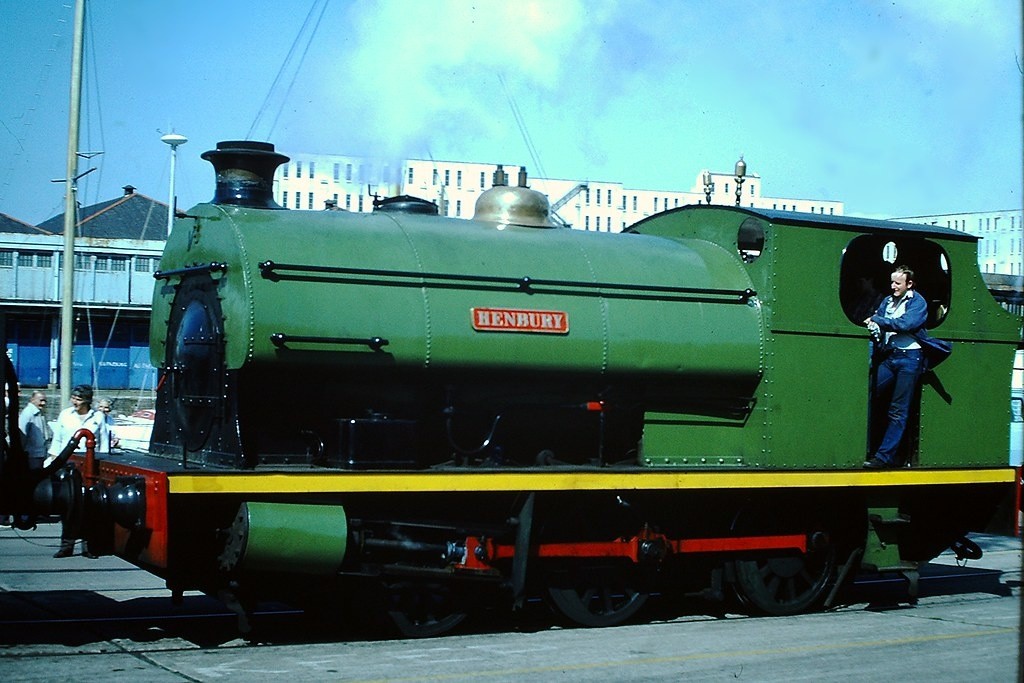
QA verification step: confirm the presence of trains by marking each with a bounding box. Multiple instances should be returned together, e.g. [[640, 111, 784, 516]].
[[35, 139, 1024, 650]]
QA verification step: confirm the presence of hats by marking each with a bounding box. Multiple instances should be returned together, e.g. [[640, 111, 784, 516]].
[[72, 384, 93, 401]]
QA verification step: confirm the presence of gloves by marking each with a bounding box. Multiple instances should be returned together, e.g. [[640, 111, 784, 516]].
[[867, 321, 881, 341]]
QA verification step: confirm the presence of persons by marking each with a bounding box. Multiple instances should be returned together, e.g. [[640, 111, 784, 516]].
[[864, 265, 952, 467], [95, 397, 115, 424], [47, 384, 109, 559], [18, 390, 52, 503]]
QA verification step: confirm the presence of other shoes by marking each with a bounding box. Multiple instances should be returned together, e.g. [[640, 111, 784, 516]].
[[81, 551, 98, 558], [863, 456, 891, 470], [53, 549, 70, 557]]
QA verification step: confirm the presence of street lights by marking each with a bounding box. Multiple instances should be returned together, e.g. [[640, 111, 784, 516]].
[[160, 128, 191, 238]]
